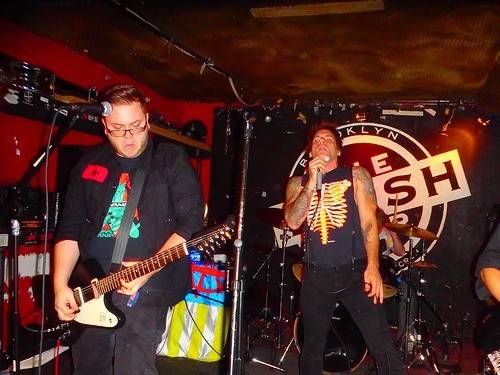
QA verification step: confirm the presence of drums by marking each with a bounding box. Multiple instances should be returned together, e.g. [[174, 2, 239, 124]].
[[378, 254, 399, 298], [293, 301, 368, 374]]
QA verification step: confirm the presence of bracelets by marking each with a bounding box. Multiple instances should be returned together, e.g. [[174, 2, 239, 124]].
[[300, 186, 315, 193]]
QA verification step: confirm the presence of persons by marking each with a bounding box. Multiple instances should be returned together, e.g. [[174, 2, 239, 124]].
[[474, 226, 500, 353], [283, 121, 405, 375], [50, 82, 205, 375]]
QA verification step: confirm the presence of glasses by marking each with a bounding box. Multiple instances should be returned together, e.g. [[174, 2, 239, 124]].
[[104, 112, 147, 137]]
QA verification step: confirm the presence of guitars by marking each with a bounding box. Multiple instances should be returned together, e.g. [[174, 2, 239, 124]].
[[31, 215, 237, 346]]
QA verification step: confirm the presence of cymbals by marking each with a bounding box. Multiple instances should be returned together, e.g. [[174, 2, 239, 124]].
[[400, 260, 437, 268], [258, 208, 304, 231], [384, 223, 438, 239]]
